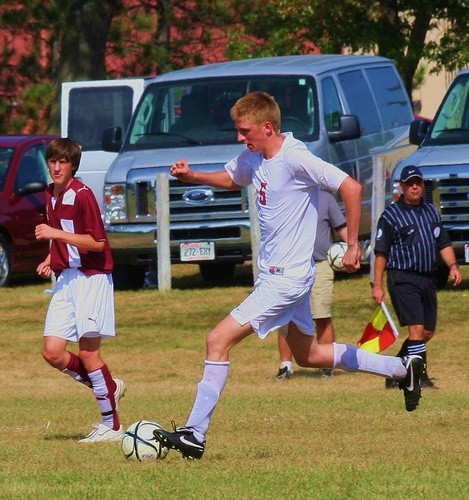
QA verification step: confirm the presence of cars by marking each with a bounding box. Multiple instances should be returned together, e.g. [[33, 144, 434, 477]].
[[0, 134, 61, 288]]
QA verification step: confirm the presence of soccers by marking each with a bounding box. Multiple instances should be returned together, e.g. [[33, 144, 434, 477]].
[[121, 422, 169, 462], [327, 241, 348, 271]]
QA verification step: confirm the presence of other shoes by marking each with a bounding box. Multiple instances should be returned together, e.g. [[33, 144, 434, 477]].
[[421, 378, 439, 389], [386, 378, 397, 388], [321, 369, 332, 378], [276, 366, 293, 380]]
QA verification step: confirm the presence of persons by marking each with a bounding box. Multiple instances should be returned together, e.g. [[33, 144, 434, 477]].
[[34, 139, 128, 443], [369, 165, 463, 389], [153, 90, 425, 464], [275, 189, 348, 379]]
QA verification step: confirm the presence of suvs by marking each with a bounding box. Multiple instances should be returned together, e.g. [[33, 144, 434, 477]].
[[389, 65, 469, 290]]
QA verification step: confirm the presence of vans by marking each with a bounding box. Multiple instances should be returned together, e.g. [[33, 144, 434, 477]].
[[61, 54, 416, 290]]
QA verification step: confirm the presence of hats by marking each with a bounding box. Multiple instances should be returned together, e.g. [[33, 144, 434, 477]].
[[400, 166, 423, 182]]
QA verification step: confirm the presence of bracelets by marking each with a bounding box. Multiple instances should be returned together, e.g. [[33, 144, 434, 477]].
[[449, 262, 459, 269]]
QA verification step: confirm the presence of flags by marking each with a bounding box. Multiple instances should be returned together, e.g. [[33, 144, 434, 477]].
[[357, 301, 400, 354]]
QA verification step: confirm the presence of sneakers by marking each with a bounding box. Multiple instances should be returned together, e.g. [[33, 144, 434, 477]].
[[153, 420, 206, 461], [397, 355, 427, 412], [112, 379, 127, 411], [79, 423, 125, 443]]
